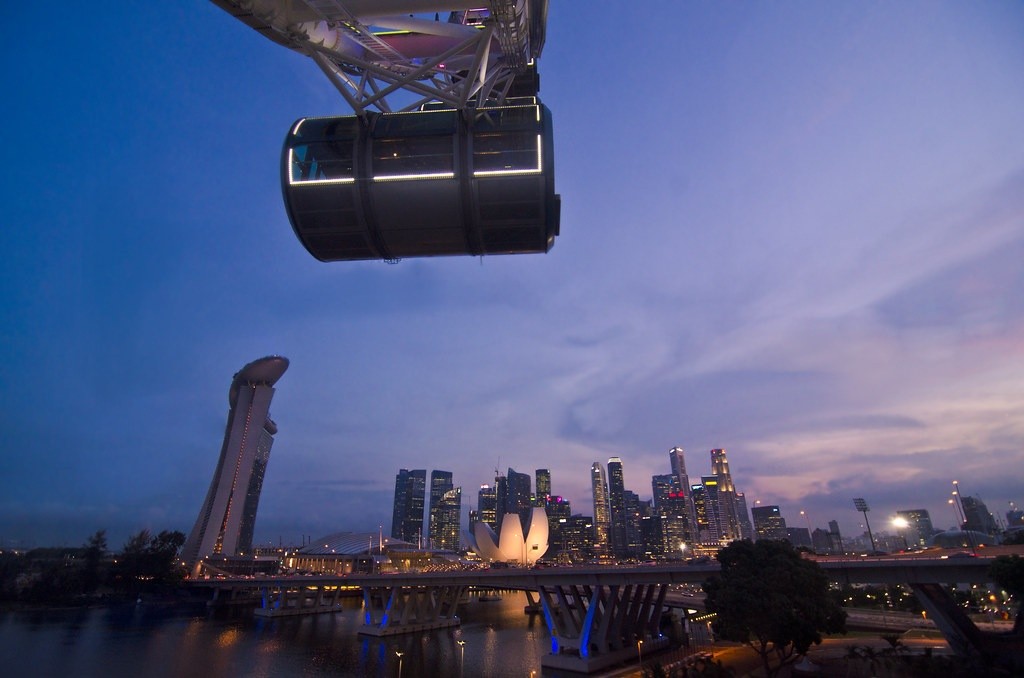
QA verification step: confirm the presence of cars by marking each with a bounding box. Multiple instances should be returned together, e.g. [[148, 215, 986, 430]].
[[681, 591, 693, 597]]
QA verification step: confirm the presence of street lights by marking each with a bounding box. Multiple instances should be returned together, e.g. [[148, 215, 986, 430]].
[[954, 479, 980, 550], [368, 536, 373, 556], [418, 527, 420, 549], [638, 641, 644, 675], [379, 525, 383, 556], [949, 499, 970, 548], [706, 622, 716, 659], [952, 491, 977, 556]]
